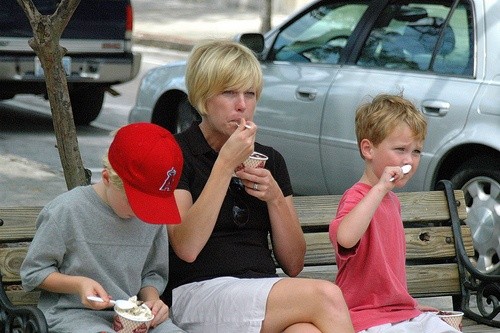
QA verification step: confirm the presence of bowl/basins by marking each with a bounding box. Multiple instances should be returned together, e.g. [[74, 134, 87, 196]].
[[433, 311, 464, 330], [233, 151, 269, 174], [114, 305, 154, 333]]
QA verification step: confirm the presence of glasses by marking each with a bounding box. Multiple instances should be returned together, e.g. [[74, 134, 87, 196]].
[[227, 176, 250, 229]]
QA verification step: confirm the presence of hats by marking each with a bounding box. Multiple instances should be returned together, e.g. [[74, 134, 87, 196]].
[[108, 123, 183, 225]]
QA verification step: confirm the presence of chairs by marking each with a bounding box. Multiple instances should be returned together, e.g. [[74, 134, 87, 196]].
[[402, 16, 455, 69]]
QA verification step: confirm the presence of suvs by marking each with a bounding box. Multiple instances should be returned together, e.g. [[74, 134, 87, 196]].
[[0, 0, 141, 125], [128, 0, 500, 279]]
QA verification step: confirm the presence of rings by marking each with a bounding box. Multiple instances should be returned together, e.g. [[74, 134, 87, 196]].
[[254, 183, 258, 190]]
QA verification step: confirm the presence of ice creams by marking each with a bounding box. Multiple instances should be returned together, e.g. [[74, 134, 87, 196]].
[[435, 310, 454, 315], [250, 152, 266, 158], [115, 295, 154, 320]]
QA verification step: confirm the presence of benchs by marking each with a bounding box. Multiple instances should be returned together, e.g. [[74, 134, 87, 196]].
[[0, 180, 500, 333]]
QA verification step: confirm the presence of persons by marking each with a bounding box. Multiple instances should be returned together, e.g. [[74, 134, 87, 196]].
[[162, 40, 353, 333], [19, 122, 188, 333], [330, 94, 461, 333]]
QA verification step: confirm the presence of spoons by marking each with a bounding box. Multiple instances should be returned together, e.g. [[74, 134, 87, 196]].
[[387, 165, 413, 182], [86, 297, 136, 309]]
